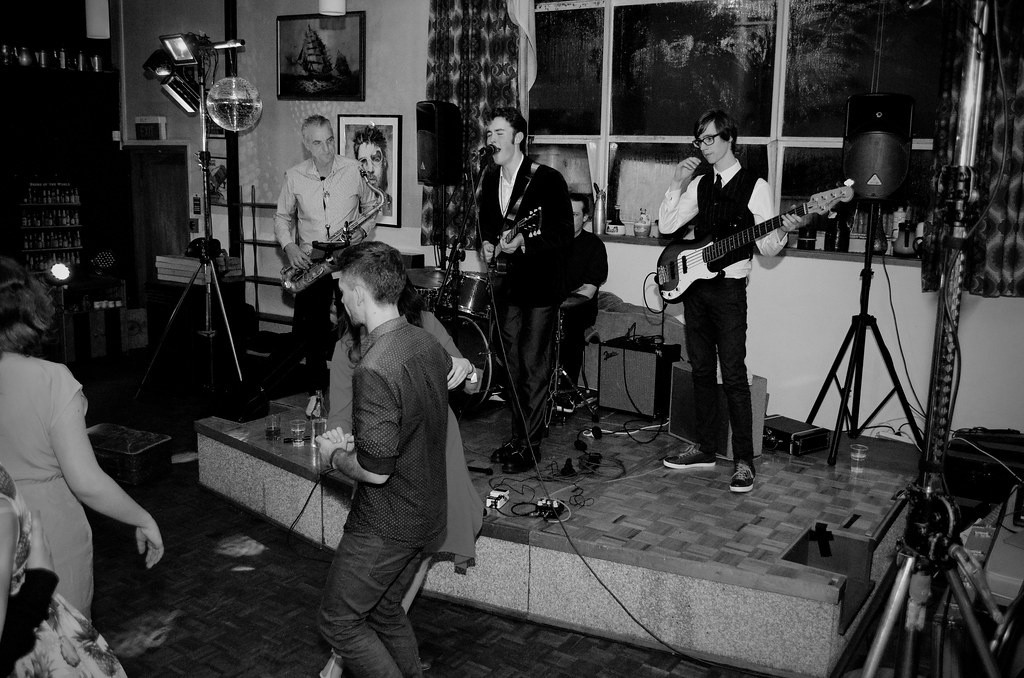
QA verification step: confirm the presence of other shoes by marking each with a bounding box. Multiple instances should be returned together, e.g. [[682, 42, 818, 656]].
[[304, 396, 322, 420]]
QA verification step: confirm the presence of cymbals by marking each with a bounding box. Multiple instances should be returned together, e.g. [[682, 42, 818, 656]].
[[405, 267, 450, 290], [560, 292, 590, 308]]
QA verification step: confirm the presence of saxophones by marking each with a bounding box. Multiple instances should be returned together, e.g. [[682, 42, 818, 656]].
[[279, 168, 389, 295]]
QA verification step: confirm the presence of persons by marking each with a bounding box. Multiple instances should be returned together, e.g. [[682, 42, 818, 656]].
[[658, 108, 807, 494], [353, 126, 392, 221], [316, 244, 453, 677], [275, 115, 381, 403], [0, 256, 164, 623], [317, 247, 482, 678], [0, 511, 59, 678], [474, 107, 578, 474], [0, 465, 128, 677], [556, 190, 609, 411]]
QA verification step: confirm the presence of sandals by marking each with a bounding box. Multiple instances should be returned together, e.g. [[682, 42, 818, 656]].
[[419, 658, 431, 671], [319, 648, 343, 678]]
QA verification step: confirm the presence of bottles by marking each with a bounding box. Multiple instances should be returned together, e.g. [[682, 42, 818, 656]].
[[604, 205, 626, 236], [633, 207, 651, 238], [18, 186, 83, 273], [592, 190, 606, 235]]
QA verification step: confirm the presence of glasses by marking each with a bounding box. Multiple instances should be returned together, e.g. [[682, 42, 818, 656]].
[[692, 133, 719, 148]]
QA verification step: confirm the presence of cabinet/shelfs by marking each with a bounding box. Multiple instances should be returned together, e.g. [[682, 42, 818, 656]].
[[51, 280, 130, 366], [144, 279, 245, 382], [238, 184, 293, 326], [18, 202, 83, 274]]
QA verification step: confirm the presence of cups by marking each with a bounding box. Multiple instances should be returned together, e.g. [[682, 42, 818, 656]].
[[264, 415, 281, 444], [850, 444, 868, 474], [289, 419, 306, 447]]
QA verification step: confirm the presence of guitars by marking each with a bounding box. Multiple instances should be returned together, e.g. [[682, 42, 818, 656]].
[[484, 206, 545, 295], [655, 178, 856, 305]]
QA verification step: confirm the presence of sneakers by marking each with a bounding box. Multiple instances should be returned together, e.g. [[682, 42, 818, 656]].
[[502, 443, 541, 474], [490, 438, 517, 463], [729, 459, 755, 492], [663, 444, 716, 468]]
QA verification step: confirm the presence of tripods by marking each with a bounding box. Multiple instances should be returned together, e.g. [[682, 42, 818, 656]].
[[806, 202, 923, 466], [542, 311, 601, 437]]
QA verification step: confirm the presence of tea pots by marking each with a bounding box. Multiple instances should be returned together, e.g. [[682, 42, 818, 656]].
[[0, 44, 103, 72]]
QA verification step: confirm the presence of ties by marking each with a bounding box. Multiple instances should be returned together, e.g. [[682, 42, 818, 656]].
[[713, 173, 723, 191]]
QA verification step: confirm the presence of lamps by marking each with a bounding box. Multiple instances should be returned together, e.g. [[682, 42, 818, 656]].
[[158, 33, 199, 66], [319, 0, 346, 16], [85, 0, 110, 39]]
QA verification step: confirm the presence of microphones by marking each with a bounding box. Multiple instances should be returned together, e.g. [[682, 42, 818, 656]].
[[473, 144, 496, 157]]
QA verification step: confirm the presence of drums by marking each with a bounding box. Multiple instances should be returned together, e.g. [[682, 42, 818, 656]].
[[435, 314, 494, 414], [433, 272, 494, 321]]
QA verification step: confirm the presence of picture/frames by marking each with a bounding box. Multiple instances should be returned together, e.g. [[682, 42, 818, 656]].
[[337, 114, 403, 228], [276, 10, 366, 101]]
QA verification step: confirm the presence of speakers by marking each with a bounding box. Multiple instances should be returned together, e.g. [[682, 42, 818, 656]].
[[842, 93, 915, 203], [668, 360, 768, 461], [416, 100, 463, 186], [598, 336, 681, 422]]
